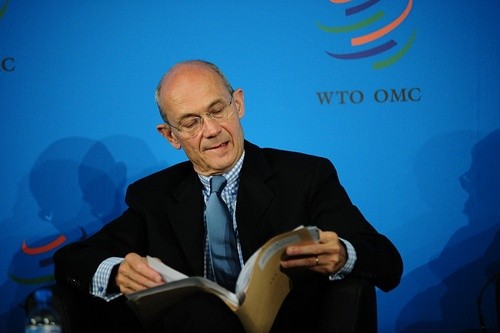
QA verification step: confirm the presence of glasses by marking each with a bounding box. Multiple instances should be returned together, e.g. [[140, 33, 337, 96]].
[[167, 94, 233, 134]]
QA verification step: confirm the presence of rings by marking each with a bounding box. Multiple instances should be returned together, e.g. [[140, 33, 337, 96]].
[[316, 255, 318, 265]]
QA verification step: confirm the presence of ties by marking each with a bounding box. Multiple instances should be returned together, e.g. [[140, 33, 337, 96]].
[[203, 176, 241, 291]]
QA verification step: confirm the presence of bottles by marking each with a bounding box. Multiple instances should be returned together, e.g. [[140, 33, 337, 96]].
[[25, 290, 61, 333]]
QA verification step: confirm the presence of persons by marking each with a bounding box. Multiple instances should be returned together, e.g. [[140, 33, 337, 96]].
[[54, 60, 403, 333]]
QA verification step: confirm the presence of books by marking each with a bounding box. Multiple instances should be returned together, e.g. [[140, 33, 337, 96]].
[[127, 225, 319, 333]]
